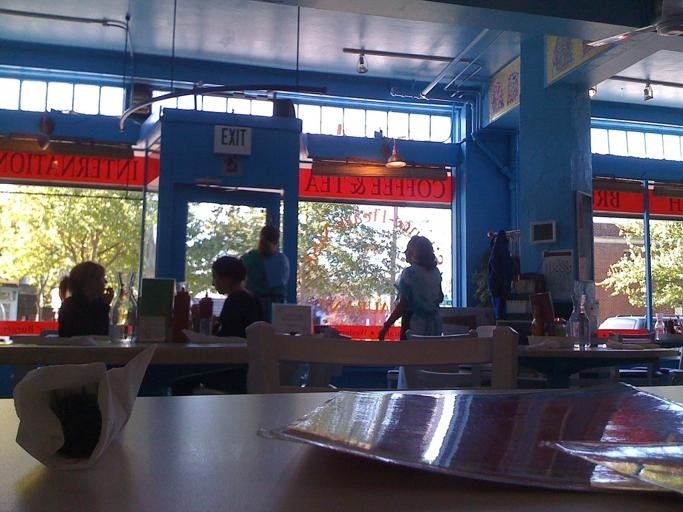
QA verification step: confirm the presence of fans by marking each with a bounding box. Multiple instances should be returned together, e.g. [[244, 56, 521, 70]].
[[587, 0, 683, 49]]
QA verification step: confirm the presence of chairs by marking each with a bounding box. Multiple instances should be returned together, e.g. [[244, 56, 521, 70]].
[[246, 319, 519, 392], [403, 327, 483, 389]]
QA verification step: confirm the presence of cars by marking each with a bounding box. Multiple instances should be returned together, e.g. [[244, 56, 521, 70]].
[[598, 314, 683, 336]]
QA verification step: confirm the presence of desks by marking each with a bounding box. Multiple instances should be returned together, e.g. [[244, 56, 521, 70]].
[[0, 384, 683, 511], [0, 340, 344, 397], [518, 343, 683, 388]]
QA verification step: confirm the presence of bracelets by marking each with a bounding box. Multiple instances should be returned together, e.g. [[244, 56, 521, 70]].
[[383, 321, 390, 328]]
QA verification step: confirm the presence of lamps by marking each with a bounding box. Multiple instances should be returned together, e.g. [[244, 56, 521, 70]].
[[37, 116, 53, 152], [642, 81, 655, 102], [355, 50, 369, 76], [384, 138, 407, 169]]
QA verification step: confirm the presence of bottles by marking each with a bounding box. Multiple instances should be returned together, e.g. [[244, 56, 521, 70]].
[[192, 302, 199, 333], [654, 312, 665, 343], [531, 305, 543, 337], [173, 287, 190, 342], [109, 270, 140, 344], [566, 294, 591, 349], [198, 293, 214, 341]]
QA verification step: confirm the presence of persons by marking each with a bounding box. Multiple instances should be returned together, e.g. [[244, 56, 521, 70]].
[[211, 256, 267, 393], [56, 261, 113, 336], [240, 225, 290, 325], [377, 235, 444, 341]]
